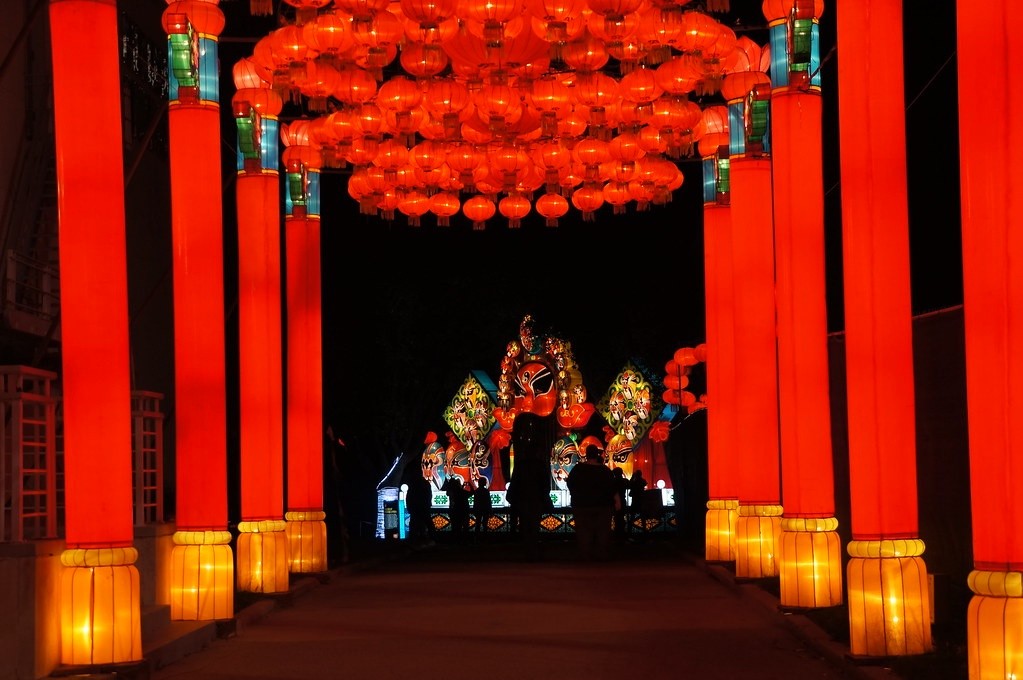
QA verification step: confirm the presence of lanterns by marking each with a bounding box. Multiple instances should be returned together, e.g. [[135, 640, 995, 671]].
[[161, 0, 825, 232], [663, 344, 707, 414]]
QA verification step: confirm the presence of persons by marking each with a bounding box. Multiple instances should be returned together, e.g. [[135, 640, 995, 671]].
[[403, 445, 651, 564]]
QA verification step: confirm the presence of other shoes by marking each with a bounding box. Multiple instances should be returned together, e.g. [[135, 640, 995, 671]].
[[416, 544, 426, 551], [426, 540, 436, 548]]
